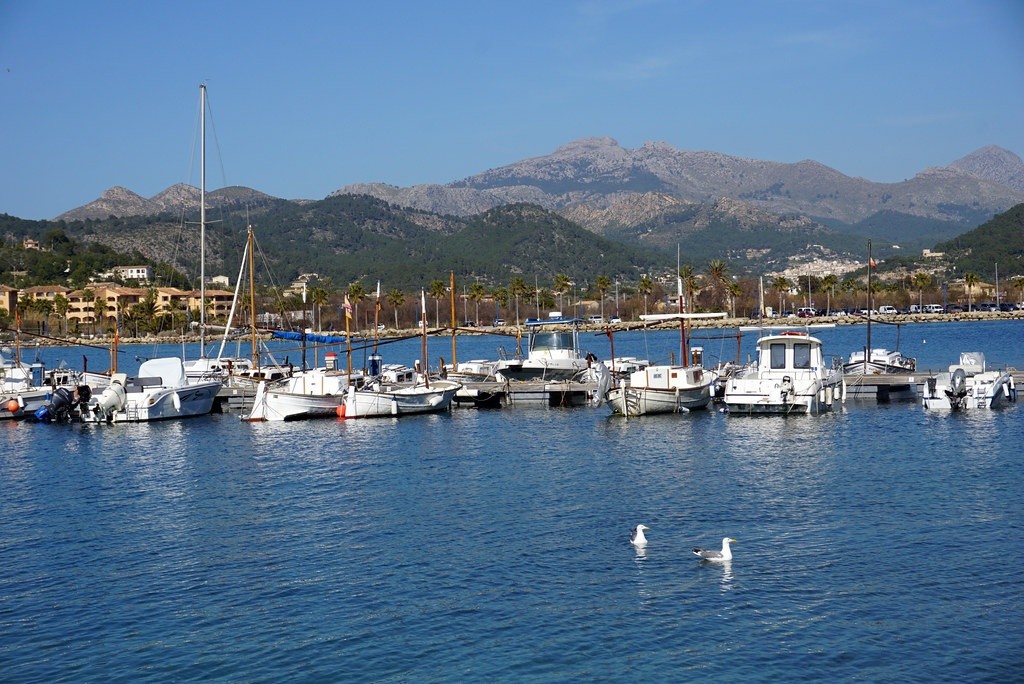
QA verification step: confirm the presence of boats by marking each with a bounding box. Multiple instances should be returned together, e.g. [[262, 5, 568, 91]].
[[0, 85, 1021, 426]]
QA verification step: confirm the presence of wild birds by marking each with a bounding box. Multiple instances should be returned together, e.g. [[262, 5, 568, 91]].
[[628, 524, 650, 547], [692, 536, 737, 562]]
